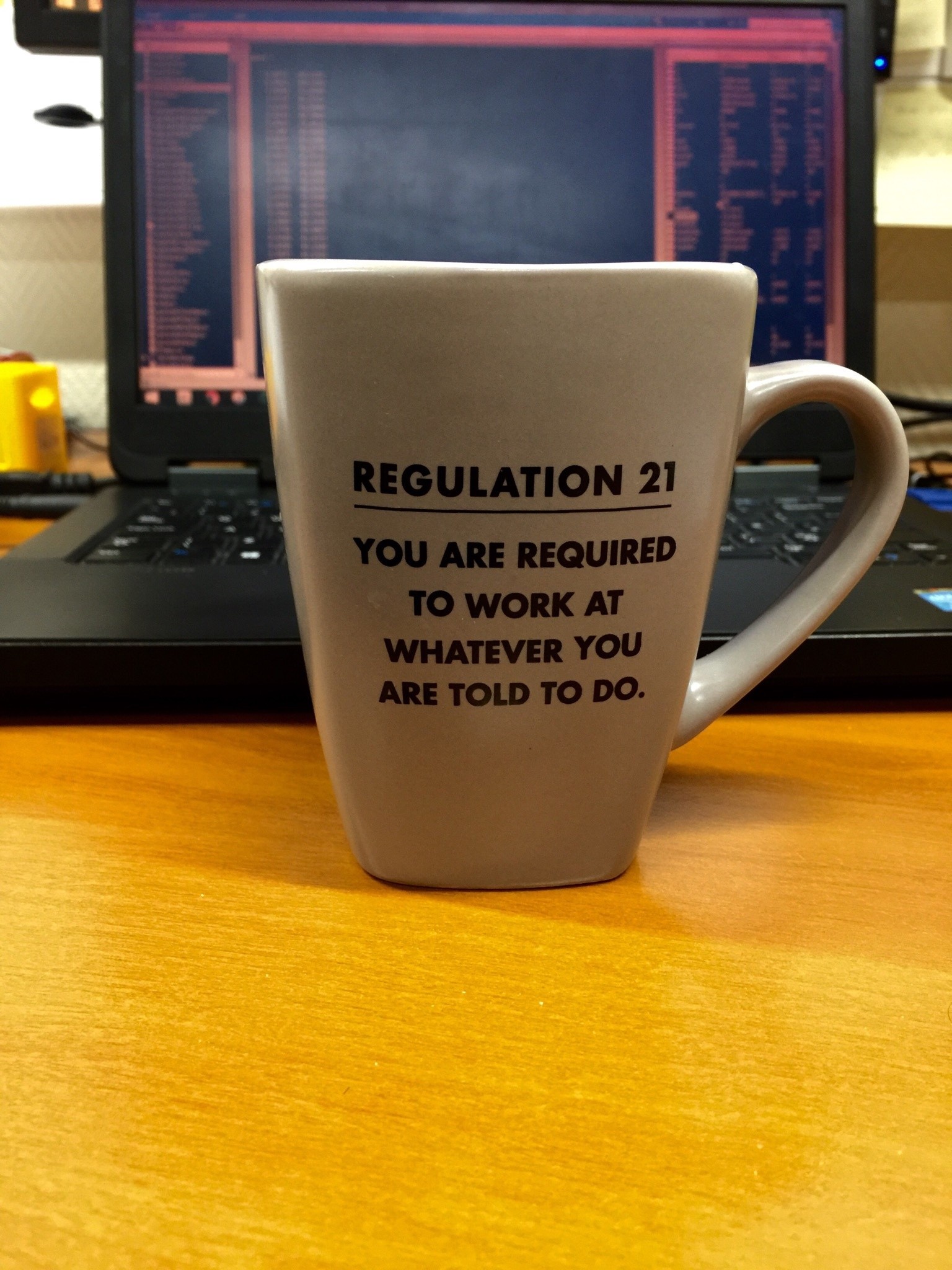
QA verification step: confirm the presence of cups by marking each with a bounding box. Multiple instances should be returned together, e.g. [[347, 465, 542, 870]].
[[255, 258, 911, 899]]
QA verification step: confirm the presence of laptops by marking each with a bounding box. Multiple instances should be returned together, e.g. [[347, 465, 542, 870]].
[[1, 0, 952, 722]]
